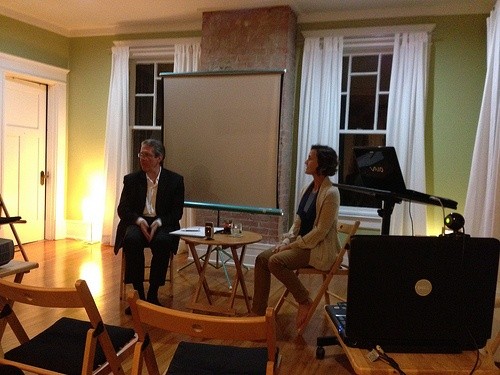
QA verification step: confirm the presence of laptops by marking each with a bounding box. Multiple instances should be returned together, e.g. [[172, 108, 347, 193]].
[[353, 146, 458, 205], [325, 234, 500, 350]]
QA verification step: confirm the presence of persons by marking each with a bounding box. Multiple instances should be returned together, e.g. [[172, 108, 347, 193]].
[[112, 138, 185, 314], [238, 145, 342, 332]]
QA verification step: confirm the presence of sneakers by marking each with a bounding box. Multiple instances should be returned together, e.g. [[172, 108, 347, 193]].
[[126, 298, 161, 316]]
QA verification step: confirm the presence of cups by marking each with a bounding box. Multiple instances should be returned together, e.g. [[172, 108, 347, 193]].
[[231, 223, 242, 237]]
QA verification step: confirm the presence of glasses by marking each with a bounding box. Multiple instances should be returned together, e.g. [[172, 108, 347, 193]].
[[138, 153, 155, 160]]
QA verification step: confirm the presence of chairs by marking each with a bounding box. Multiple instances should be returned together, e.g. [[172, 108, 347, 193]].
[[119, 247, 174, 302], [0, 278, 140, 375], [126, 289, 283, 375], [274, 219, 360, 336]]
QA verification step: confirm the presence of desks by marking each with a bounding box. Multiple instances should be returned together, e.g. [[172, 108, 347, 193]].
[[322, 303, 500, 375], [181, 227, 263, 314], [0, 259, 39, 279]]
